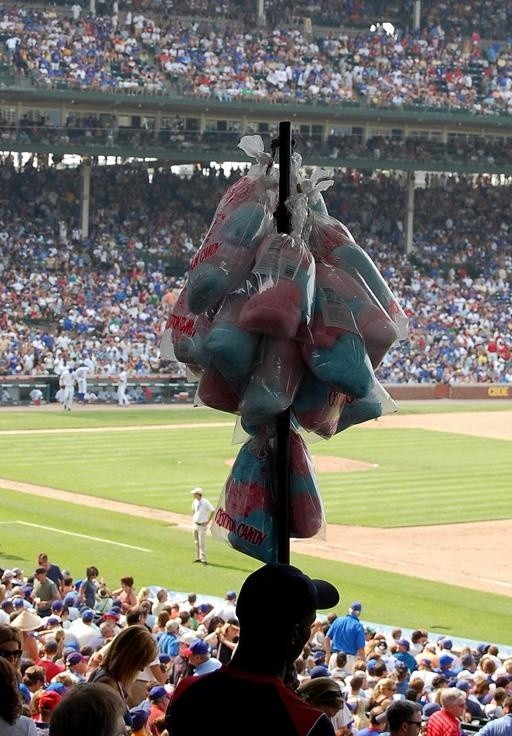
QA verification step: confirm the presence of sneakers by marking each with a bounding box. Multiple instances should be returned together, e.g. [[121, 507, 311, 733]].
[[192, 559, 208, 565]]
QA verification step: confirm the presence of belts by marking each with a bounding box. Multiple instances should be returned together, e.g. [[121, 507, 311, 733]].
[[194, 521, 201, 526]]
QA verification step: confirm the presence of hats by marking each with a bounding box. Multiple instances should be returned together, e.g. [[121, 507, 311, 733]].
[[236, 564, 339, 618], [149, 686, 171, 701], [129, 706, 150, 730], [310, 665, 333, 679], [18, 640, 90, 712], [349, 601, 361, 610], [344, 626, 476, 723], [2, 567, 121, 625], [158, 601, 211, 663], [226, 591, 236, 598], [191, 487, 203, 496], [314, 649, 327, 661]]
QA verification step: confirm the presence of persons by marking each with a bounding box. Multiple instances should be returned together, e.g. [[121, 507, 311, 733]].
[[190, 488, 215, 563]]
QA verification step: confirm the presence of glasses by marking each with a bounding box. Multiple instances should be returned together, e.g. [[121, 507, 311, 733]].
[[0, 650, 23, 659]]
